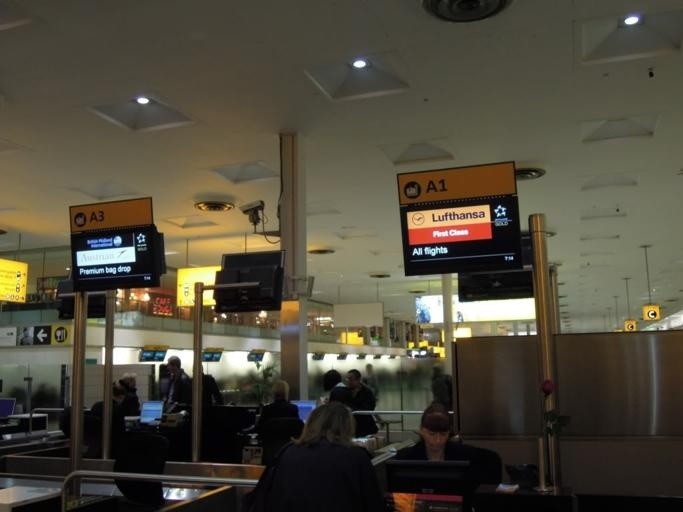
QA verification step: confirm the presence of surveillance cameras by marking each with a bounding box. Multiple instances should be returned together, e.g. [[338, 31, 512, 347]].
[[238, 200, 264, 213]]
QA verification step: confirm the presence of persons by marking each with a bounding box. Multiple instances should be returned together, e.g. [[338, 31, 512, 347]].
[[20, 328, 32, 345], [89, 356, 224, 459], [256, 380, 304, 465], [416, 296, 464, 322], [242, 400, 389, 512], [431, 365, 451, 412], [395, 402, 482, 485], [323, 364, 387, 438]]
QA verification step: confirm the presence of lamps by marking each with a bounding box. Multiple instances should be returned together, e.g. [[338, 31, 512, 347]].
[[611, 245, 660, 334]]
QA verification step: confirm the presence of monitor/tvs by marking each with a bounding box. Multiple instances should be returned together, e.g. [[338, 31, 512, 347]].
[[57, 281, 106, 319], [71, 224, 167, 291], [139, 352, 263, 362], [0, 397, 16, 418], [399, 196, 523, 277], [139, 400, 165, 426], [214, 250, 285, 314], [458, 231, 536, 298], [388, 459, 470, 499], [290, 399, 317, 424]]
